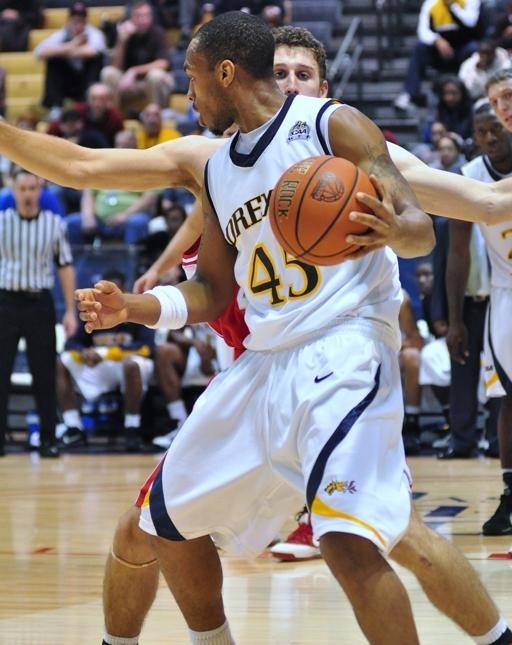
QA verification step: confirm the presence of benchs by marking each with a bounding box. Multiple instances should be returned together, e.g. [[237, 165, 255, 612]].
[[1, 2, 512, 452]]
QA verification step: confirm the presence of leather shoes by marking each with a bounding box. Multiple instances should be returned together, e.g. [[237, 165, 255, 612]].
[[124, 435, 163, 454], [39, 436, 60, 457], [437, 447, 470, 460]]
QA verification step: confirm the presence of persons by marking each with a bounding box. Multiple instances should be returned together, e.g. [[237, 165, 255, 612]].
[[442, 104, 511, 540], [261, 504, 325, 565], [71, 10, 437, 644], [2, 0, 512, 461], [0, 24, 511, 645]]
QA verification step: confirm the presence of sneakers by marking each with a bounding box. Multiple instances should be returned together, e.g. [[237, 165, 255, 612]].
[[481, 492, 512, 537], [402, 418, 421, 439], [270, 507, 329, 562], [54, 428, 90, 451], [151, 426, 179, 450], [432, 433, 452, 450]]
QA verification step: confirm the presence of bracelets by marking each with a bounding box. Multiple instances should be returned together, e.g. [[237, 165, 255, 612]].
[[140, 285, 187, 331]]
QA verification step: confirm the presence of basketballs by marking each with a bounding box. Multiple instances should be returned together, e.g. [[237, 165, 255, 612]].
[[269, 155, 381, 266]]
